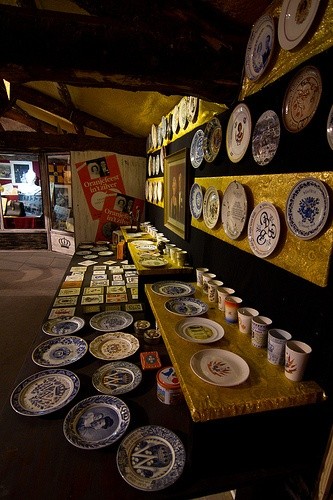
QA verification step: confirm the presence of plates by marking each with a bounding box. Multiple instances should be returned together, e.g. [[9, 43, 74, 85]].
[[42, 316, 85, 336], [164, 296, 208, 316], [91, 361, 143, 395], [277, 0, 320, 51], [125, 227, 169, 268], [63, 394, 129, 450], [75, 239, 113, 260], [117, 423, 186, 491], [174, 316, 224, 344], [9, 368, 80, 416], [88, 310, 134, 331], [31, 336, 88, 367], [245, 16, 276, 80], [88, 332, 140, 358], [189, 348, 251, 387], [145, 95, 333, 259], [150, 281, 196, 296]]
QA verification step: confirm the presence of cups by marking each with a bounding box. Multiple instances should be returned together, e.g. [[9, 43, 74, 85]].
[[197, 268, 312, 381], [141, 221, 188, 267]]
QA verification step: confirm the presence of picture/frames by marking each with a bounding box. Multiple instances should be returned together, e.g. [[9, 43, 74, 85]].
[[163, 147, 193, 241]]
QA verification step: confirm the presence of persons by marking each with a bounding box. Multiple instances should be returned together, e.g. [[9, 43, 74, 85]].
[[114, 198, 134, 214], [88, 159, 110, 179]]
